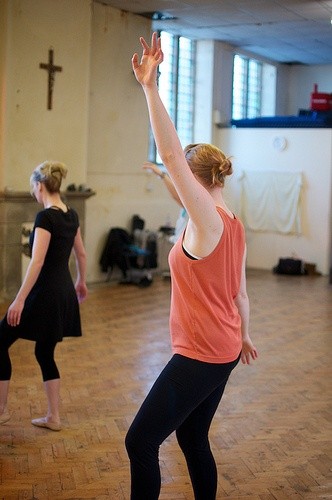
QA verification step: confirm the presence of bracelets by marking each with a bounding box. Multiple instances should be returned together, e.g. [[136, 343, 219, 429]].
[[161, 171, 166, 179]]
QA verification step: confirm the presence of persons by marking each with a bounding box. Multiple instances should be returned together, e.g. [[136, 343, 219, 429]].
[[140, 161, 189, 245], [0, 159, 89, 431], [125, 31, 259, 500]]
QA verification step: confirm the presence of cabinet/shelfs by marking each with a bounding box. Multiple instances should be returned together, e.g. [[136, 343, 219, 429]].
[[0, 191, 95, 304]]
